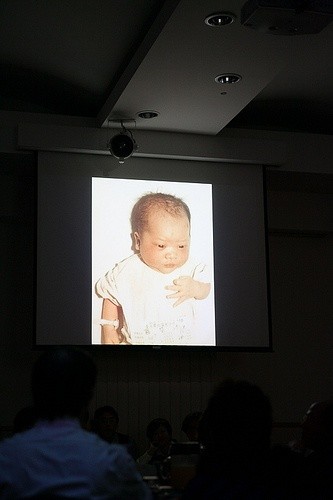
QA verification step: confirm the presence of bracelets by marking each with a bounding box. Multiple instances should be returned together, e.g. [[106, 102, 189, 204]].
[[99, 318, 119, 330]]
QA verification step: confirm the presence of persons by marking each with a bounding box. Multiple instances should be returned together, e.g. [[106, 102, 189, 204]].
[[0, 350, 151, 500], [92, 380, 333, 500], [95, 193, 216, 346]]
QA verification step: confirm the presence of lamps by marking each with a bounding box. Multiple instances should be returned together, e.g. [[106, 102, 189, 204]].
[[105, 121, 137, 160]]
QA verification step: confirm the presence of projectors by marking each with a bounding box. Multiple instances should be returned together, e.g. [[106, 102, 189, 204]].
[[240, 0, 333, 34]]
